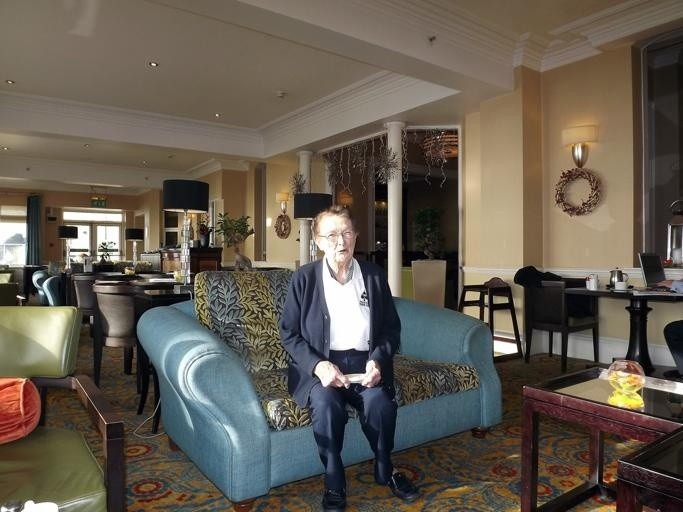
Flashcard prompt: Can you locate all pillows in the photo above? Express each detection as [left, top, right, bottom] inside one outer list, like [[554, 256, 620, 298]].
[[48, 259, 67, 276]]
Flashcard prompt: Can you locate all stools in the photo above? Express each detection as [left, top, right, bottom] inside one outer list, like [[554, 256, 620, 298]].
[[454, 283, 524, 364]]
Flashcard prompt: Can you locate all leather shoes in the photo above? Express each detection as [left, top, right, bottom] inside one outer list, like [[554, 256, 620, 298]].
[[322, 488, 347, 512], [388, 467, 421, 502]]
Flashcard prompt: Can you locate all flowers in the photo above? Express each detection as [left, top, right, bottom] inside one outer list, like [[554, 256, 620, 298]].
[[196, 210, 214, 235]]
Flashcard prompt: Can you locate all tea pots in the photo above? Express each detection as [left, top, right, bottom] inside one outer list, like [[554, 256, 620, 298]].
[[609, 267, 628, 287]]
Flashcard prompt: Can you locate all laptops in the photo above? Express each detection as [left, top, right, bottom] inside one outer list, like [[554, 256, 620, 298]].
[[637, 253, 670, 291]]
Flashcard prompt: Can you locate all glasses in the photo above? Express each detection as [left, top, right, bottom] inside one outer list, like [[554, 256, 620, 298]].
[[316, 230, 356, 239]]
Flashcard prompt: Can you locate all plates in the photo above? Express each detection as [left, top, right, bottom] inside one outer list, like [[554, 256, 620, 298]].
[[613, 290, 627, 293]]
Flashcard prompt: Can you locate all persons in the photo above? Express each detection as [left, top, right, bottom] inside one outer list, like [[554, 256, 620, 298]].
[[654, 280, 683, 381], [279, 204, 419, 511]]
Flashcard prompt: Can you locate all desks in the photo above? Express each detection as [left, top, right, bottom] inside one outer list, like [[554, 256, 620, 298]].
[[94, 277, 199, 309], [565, 284, 683, 374], [57, 271, 174, 306]]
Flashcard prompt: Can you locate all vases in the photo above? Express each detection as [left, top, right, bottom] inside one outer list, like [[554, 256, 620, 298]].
[[199, 229, 213, 246]]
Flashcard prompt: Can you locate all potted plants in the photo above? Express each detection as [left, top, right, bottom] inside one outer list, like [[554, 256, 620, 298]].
[[213, 212, 270, 271], [92, 240, 116, 266]]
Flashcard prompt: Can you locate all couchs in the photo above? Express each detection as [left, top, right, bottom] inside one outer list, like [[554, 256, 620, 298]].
[[135, 265, 504, 512]]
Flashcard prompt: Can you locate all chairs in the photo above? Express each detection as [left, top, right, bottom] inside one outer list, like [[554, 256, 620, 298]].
[[70, 275, 102, 334], [523, 280, 601, 369], [0, 305, 126, 512], [0, 269, 15, 283], [91, 264, 113, 272], [87, 279, 144, 389], [31, 269, 51, 306], [661, 318, 683, 376], [42, 275, 70, 307], [70, 263, 83, 274], [0, 283, 25, 306]]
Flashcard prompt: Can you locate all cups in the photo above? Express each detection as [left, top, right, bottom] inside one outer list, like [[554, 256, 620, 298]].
[[615, 282, 625, 290]]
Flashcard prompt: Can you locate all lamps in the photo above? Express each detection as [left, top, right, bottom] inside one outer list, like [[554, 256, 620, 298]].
[[162, 178, 209, 295], [294, 192, 336, 264], [57, 226, 78, 273], [125, 229, 144, 269], [558, 122, 598, 167], [277, 190, 290, 213]]
